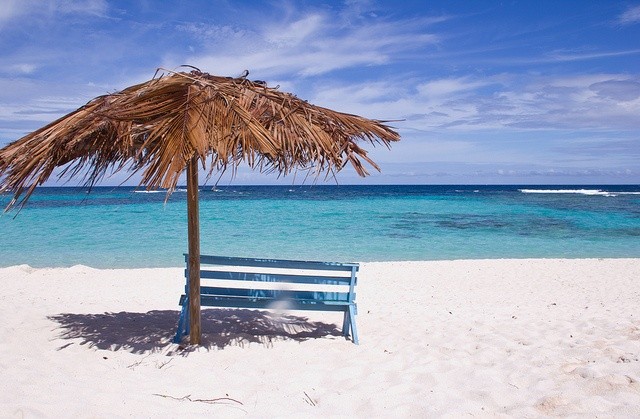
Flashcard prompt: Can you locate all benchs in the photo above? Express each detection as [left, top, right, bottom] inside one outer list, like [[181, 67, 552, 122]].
[[177, 253, 359, 345]]
